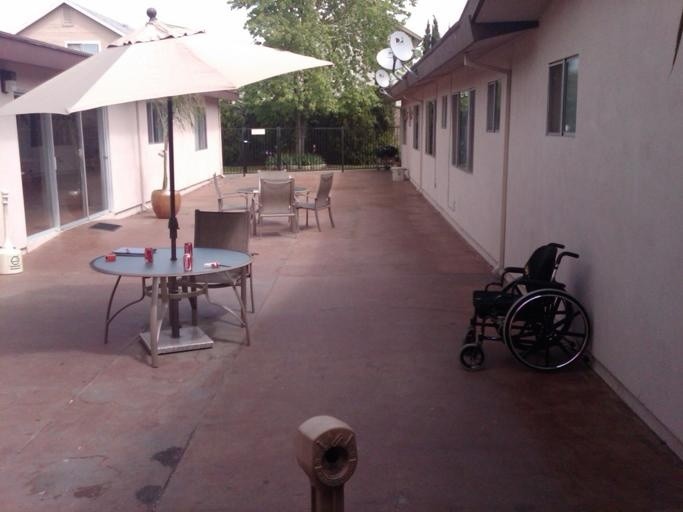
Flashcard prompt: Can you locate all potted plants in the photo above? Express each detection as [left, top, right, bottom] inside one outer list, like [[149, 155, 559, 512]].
[[149, 94, 205, 218]]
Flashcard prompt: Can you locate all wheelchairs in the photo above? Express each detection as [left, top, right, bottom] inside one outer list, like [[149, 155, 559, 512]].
[[456, 242, 592, 374]]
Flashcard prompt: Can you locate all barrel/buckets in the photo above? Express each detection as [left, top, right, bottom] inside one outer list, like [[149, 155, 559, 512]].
[[391, 167, 407, 181]]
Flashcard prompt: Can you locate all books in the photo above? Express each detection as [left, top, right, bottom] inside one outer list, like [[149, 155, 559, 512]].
[[111, 246, 155, 257]]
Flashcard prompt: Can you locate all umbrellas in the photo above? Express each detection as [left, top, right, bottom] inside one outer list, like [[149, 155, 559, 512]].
[[0, 6, 333, 339]]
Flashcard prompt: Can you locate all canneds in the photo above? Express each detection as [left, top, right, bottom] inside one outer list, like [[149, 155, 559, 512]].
[[144, 247, 153, 264], [184, 242, 192, 255], [183, 254, 192, 272]]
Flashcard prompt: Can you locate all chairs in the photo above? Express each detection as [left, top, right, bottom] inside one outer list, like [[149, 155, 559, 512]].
[[169, 209, 254, 346], [213, 168, 334, 235]]
